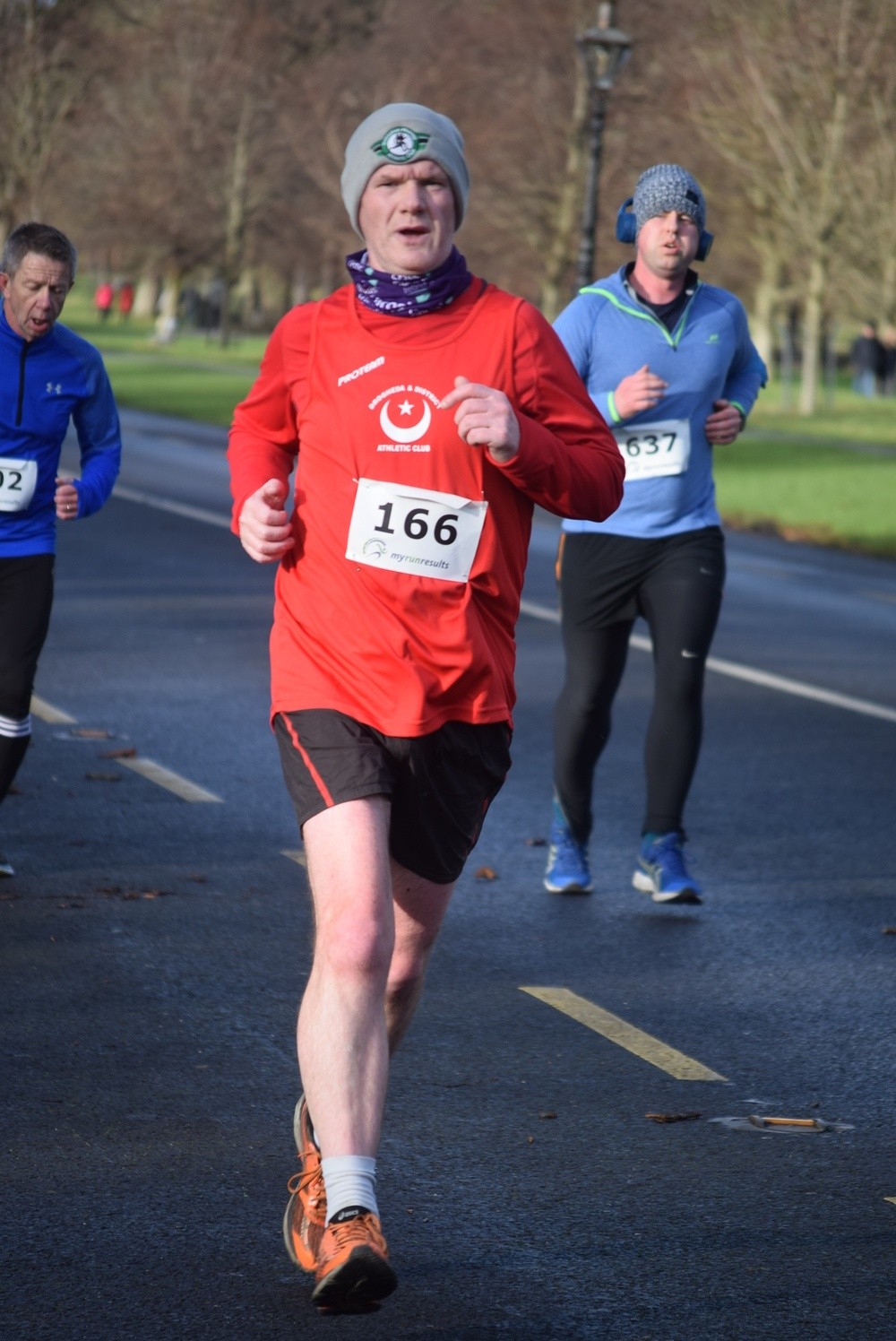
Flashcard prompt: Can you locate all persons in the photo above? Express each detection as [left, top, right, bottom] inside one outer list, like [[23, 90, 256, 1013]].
[[857, 319, 883, 397], [222, 104, 630, 1315], [119, 279, 134, 327], [94, 277, 114, 329], [542, 162, 771, 902], [0, 220, 126, 874]]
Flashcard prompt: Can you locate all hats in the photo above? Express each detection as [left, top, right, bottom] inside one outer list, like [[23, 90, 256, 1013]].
[[341, 103, 468, 238], [632, 164, 707, 237]]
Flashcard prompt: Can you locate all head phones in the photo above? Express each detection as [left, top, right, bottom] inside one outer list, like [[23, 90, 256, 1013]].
[[615, 198, 714, 261]]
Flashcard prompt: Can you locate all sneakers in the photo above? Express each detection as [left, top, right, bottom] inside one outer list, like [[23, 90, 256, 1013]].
[[311, 1213, 399, 1316], [283, 1092, 327, 1273], [543, 822, 594, 892], [631, 831, 703, 903]]
